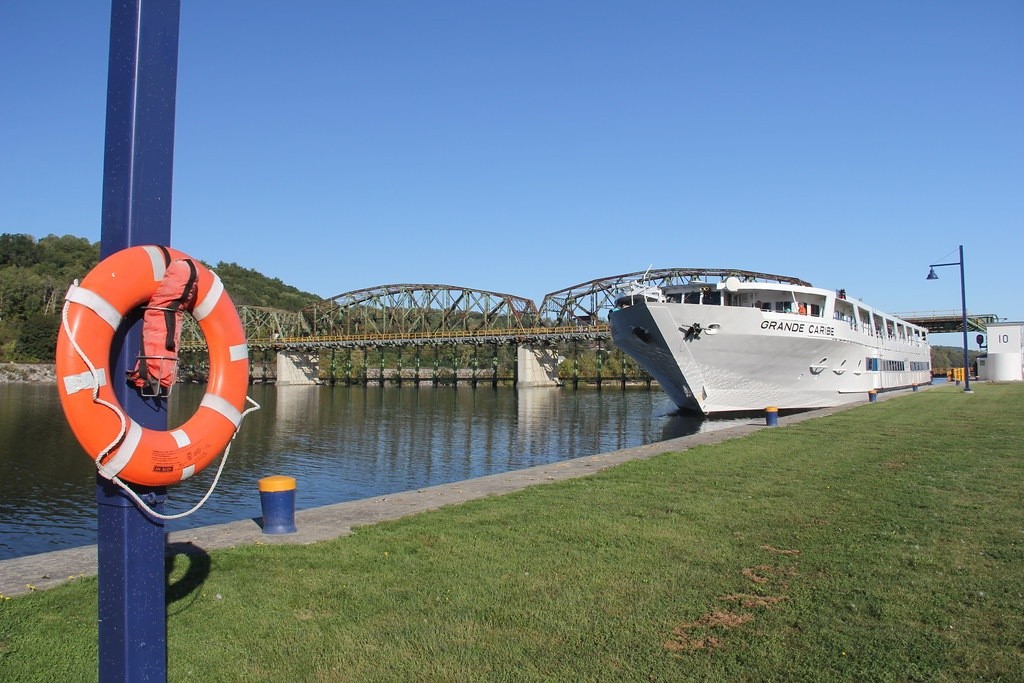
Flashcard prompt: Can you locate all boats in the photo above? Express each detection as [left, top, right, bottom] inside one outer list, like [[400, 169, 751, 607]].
[[608, 263, 933, 422]]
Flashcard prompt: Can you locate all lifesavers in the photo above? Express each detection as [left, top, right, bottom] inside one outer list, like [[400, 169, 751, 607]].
[[49, 243, 251, 490]]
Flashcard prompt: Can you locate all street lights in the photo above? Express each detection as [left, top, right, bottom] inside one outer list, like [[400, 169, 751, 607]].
[[926, 245, 974, 394]]
